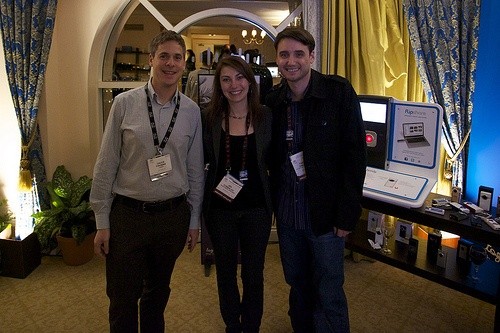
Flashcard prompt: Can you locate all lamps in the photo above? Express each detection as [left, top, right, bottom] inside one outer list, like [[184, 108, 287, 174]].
[[241, 29, 266, 45]]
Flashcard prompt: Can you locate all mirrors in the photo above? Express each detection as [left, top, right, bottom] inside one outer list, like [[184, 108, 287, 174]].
[[102, 0, 304, 130]]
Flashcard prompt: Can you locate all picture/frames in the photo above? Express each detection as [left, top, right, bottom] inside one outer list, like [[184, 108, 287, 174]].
[[197, 73, 263, 111]]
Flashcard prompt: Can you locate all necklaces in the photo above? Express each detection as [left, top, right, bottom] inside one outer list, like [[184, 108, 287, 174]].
[[230, 114, 247, 119]]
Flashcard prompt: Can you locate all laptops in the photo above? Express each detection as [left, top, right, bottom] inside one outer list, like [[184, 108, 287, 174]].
[[402, 123, 430, 148]]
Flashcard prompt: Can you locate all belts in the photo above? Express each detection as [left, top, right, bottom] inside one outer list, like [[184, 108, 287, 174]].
[[114, 194, 186, 214]]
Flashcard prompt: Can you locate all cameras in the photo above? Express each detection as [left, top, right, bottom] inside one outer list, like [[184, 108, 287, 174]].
[[436, 249, 448, 266], [375, 227, 385, 245], [371, 217, 378, 228], [400, 225, 406, 238], [459, 245, 467, 260], [408, 238, 418, 262], [425, 187, 500, 231]]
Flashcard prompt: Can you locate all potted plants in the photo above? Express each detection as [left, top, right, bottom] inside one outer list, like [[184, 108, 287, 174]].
[[29, 165, 96, 266]]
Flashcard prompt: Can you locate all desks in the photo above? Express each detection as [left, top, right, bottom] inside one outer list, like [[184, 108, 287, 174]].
[[344, 192, 500, 333]]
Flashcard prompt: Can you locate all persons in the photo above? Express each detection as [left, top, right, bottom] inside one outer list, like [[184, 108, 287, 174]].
[[269, 27, 367, 333], [185, 43, 262, 105], [89, 29, 203, 333], [202, 56, 280, 333]]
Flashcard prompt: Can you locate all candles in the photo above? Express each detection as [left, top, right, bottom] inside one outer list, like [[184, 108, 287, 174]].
[[238, 48, 242, 57], [215, 52, 218, 63], [207, 47, 211, 65], [246, 53, 249, 63]]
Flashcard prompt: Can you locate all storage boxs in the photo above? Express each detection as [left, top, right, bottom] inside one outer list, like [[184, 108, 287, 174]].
[[395, 219, 414, 244], [367, 210, 385, 233]]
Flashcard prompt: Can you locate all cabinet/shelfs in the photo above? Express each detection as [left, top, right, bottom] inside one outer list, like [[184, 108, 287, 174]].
[[114, 47, 151, 81]]
